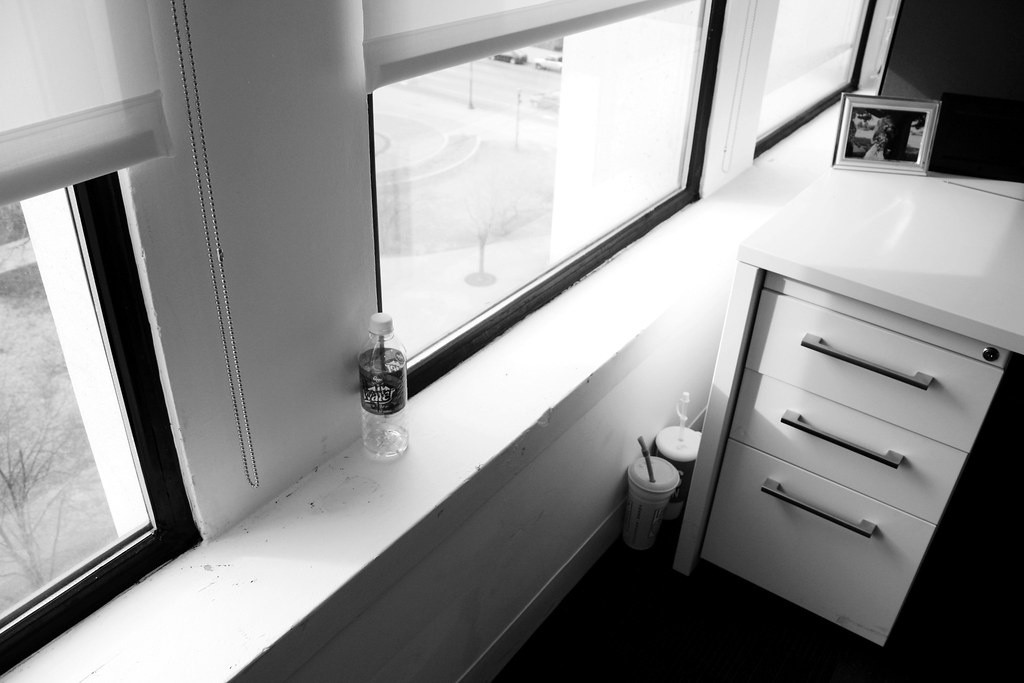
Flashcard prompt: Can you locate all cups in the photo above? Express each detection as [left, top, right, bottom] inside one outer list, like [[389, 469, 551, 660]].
[[624, 457, 680, 550], [656, 425, 701, 520]]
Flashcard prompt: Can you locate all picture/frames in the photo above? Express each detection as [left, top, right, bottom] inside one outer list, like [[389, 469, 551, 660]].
[[829, 92, 942, 178]]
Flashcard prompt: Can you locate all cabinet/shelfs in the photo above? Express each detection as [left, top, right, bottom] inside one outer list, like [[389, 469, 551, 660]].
[[669, 160, 1024, 647]]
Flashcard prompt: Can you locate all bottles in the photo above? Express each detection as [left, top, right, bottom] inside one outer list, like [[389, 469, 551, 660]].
[[359, 312, 407, 459]]
